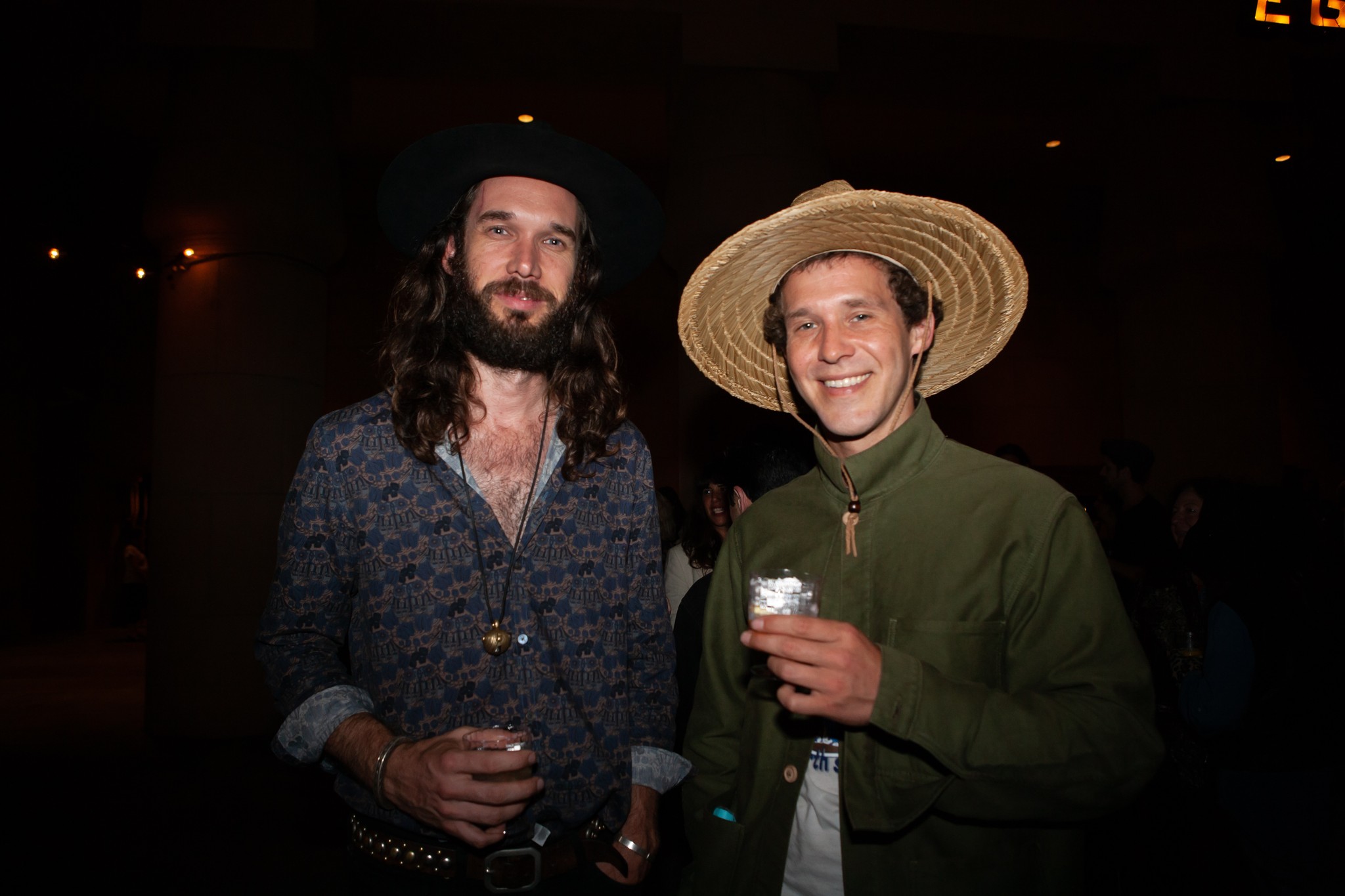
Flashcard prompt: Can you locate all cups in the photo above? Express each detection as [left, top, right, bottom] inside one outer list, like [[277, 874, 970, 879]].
[[461, 722, 535, 833], [749, 566, 822, 625]]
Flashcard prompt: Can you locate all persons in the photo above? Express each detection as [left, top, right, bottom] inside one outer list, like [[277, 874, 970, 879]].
[[641, 450, 811, 740], [254, 121, 694, 896], [119, 529, 149, 642], [993, 435, 1319, 850], [678, 178, 1165, 896]]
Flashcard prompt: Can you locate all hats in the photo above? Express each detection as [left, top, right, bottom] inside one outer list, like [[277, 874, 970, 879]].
[[677, 179, 1031, 414], [376, 121, 665, 298]]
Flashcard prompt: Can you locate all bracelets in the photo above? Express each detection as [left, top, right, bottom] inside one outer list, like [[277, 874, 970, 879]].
[[372, 735, 415, 810], [613, 833, 656, 864]]
